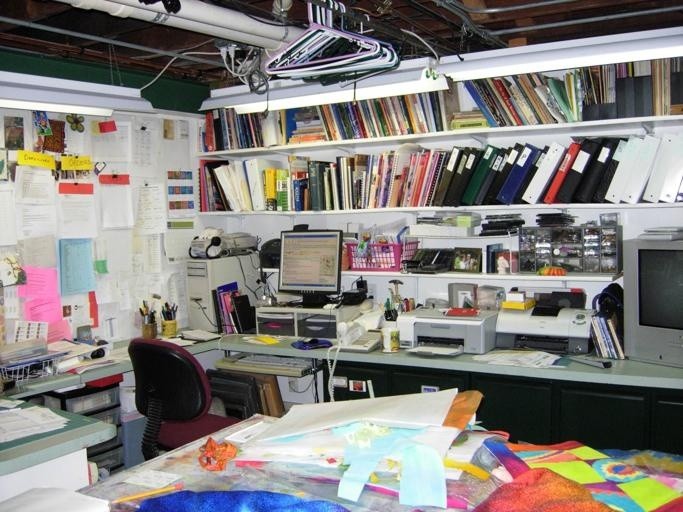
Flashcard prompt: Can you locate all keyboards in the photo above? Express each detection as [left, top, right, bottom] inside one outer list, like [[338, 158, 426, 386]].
[[237, 354, 315, 370]]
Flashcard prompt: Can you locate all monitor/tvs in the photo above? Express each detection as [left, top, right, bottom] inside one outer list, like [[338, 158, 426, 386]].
[[623, 239, 683, 368], [279, 229, 342, 307]]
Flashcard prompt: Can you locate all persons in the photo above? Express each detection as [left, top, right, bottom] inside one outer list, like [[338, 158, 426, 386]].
[[497, 255, 509, 274], [460, 254, 477, 270]]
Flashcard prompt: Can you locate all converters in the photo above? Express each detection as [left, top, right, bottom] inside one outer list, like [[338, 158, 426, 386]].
[[356, 280, 368, 292]]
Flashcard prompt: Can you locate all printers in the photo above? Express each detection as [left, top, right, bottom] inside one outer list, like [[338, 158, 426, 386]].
[[396, 308, 498, 356], [494, 291, 596, 355]]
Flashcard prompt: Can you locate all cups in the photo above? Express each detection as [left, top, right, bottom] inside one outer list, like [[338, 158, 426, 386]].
[[380, 320, 400, 353], [141, 324, 156, 340], [160, 320, 176, 339]]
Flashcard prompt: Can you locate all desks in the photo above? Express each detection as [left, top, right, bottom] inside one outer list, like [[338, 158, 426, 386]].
[[0, 328, 683, 502], [75, 413, 279, 512]]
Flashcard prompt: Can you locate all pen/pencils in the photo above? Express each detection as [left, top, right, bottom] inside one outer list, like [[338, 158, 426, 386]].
[[138, 298, 157, 324], [161, 301, 179, 321]]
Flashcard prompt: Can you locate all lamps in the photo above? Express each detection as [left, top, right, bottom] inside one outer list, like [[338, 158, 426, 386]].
[[0, 71, 159, 117]]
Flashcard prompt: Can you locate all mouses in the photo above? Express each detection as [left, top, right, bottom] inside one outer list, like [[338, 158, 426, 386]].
[[302, 337, 319, 344]]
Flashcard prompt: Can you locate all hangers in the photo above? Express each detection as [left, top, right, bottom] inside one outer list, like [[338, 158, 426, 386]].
[[267, 0, 399, 88]]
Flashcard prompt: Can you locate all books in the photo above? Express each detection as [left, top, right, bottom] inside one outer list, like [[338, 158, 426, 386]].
[[212, 283, 255, 334], [590, 312, 624, 361], [197, 55, 683, 153], [202, 145, 445, 210]]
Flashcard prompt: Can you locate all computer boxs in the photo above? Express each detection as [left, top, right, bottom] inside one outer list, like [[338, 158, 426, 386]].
[[186, 253, 262, 333]]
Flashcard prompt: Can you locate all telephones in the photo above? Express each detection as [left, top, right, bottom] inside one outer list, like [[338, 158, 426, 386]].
[[336, 324, 384, 352]]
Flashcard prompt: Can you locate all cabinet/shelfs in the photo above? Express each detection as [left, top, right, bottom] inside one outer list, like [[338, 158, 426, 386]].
[[197, 27, 683, 283]]
